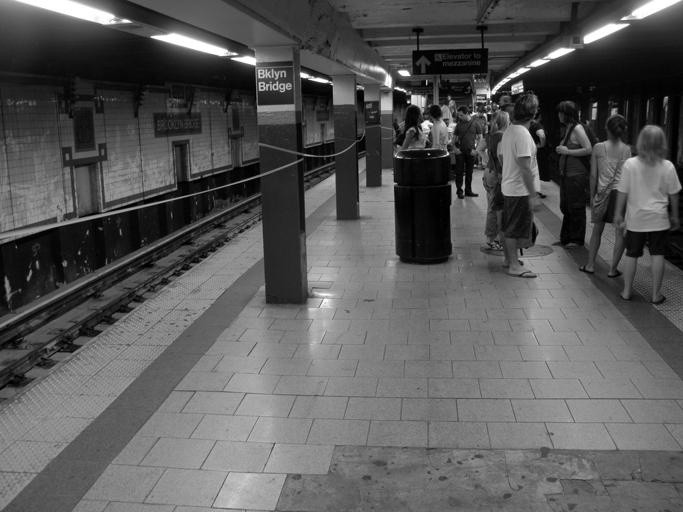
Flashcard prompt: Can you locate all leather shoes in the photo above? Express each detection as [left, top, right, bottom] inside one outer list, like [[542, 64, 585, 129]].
[[465, 192, 478, 197], [458, 193, 464, 199]]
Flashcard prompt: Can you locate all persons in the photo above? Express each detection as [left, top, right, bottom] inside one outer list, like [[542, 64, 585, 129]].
[[610, 124, 682, 304], [578, 114, 633, 278], [553, 100, 595, 251], [389, 91, 544, 280]]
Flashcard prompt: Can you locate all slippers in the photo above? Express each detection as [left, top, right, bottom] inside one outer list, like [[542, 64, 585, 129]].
[[502, 258, 523, 269], [620, 288, 632, 301], [579, 264, 595, 274], [507, 266, 537, 278], [608, 267, 621, 278], [651, 293, 666, 304]]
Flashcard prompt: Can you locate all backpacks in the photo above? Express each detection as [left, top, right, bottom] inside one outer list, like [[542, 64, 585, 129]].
[[566, 119, 601, 169]]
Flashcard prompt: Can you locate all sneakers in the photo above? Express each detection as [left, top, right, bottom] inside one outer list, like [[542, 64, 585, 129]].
[[484, 240, 504, 250]]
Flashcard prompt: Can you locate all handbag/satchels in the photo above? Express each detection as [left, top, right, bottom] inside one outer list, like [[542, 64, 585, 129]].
[[488, 184, 503, 211], [591, 192, 610, 221]]
